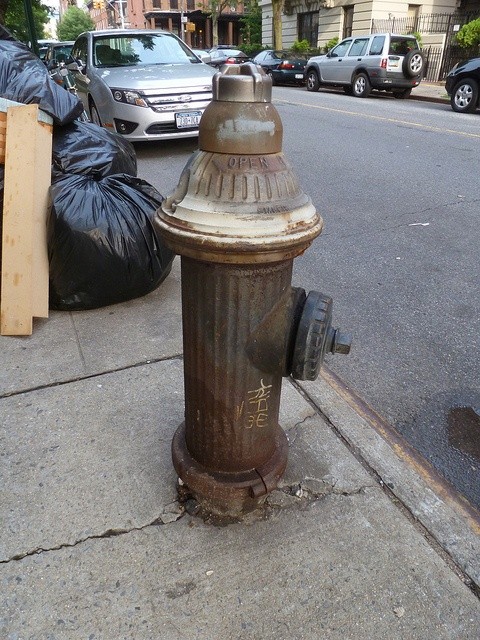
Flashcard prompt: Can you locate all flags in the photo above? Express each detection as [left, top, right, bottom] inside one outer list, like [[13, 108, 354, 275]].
[[92, 0, 106, 11]]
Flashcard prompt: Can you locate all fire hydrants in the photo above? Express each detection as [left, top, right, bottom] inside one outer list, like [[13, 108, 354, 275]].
[[152, 63, 354, 518]]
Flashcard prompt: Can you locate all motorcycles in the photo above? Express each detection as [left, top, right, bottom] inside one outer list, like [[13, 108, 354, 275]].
[[47, 53, 91, 124]]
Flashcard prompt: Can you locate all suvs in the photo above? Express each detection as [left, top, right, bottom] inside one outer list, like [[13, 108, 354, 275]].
[[302, 32, 426, 98]]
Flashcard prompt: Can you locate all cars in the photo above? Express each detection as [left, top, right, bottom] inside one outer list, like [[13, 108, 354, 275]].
[[245, 50, 309, 85], [445, 56, 480, 113], [61, 28, 220, 143], [44, 41, 81, 64], [203, 49, 251, 73]]
